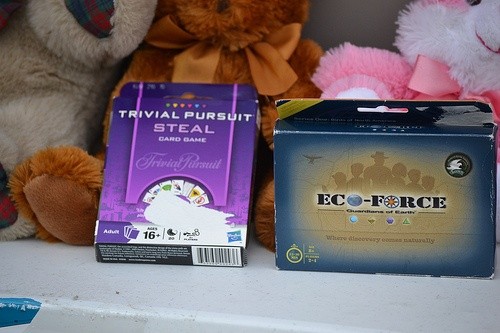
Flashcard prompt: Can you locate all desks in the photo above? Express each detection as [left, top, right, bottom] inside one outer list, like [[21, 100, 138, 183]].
[[0, 233, 500, 333]]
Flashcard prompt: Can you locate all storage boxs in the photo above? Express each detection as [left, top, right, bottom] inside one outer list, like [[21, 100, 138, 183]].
[[97, 82, 258, 268], [272, 97, 495, 278]]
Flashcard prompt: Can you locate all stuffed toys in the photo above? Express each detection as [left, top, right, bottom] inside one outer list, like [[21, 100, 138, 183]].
[[313, 0, 500, 244], [0, 0, 160, 242], [9, 0, 326, 251]]
